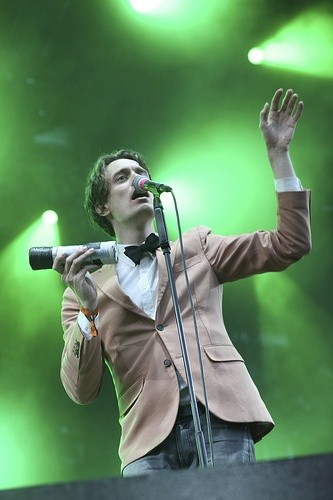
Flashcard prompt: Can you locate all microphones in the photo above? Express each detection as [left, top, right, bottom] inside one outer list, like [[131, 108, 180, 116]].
[[134, 176, 172, 194]]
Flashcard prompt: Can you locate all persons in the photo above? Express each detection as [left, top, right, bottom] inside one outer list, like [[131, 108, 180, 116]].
[[60, 88, 313, 476]]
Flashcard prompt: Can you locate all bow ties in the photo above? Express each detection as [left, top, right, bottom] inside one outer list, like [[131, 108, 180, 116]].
[[124, 233, 161, 264]]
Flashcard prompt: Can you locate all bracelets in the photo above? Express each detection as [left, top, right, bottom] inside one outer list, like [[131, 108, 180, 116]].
[[80, 307, 100, 338]]
